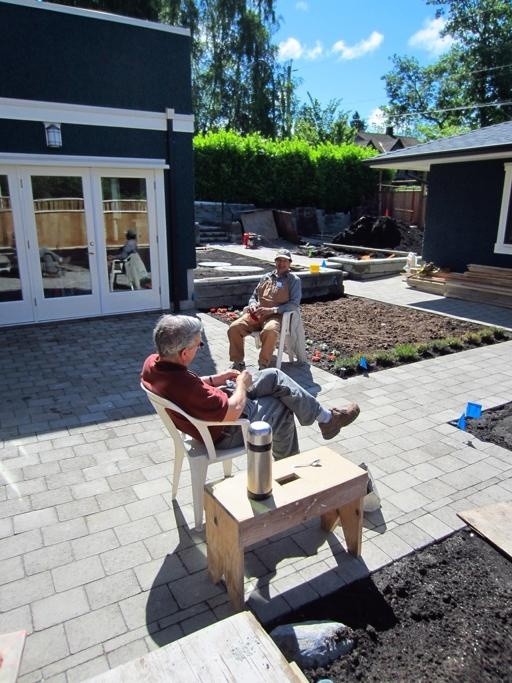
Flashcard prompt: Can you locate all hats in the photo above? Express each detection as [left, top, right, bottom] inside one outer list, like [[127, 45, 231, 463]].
[[274, 248, 293, 262]]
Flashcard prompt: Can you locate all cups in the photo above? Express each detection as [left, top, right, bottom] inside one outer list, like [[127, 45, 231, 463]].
[[309, 264, 319, 272]]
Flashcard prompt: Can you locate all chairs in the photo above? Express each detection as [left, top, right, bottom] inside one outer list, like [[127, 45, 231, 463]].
[[250, 307, 300, 369], [110, 254, 139, 291], [140, 381, 251, 532]]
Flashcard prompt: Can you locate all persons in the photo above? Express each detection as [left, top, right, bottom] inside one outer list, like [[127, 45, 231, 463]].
[[140, 313, 361, 463], [227, 248, 302, 371], [106, 229, 137, 273], [12, 232, 71, 277]]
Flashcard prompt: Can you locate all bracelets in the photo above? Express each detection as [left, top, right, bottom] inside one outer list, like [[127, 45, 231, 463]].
[[273, 307, 278, 314], [209, 375, 214, 387]]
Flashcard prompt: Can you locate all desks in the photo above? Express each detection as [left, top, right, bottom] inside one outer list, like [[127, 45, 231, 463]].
[[205, 446, 369, 610]]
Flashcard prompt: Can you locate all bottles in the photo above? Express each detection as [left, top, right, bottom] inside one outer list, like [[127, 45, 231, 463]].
[[322, 260, 327, 267], [246, 421, 273, 500]]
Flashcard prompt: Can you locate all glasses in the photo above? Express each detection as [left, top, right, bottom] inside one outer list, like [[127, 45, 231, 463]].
[[196, 340, 204, 349]]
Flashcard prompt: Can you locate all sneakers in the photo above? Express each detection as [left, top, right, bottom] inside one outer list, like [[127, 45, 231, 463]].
[[317, 402, 360, 440]]
